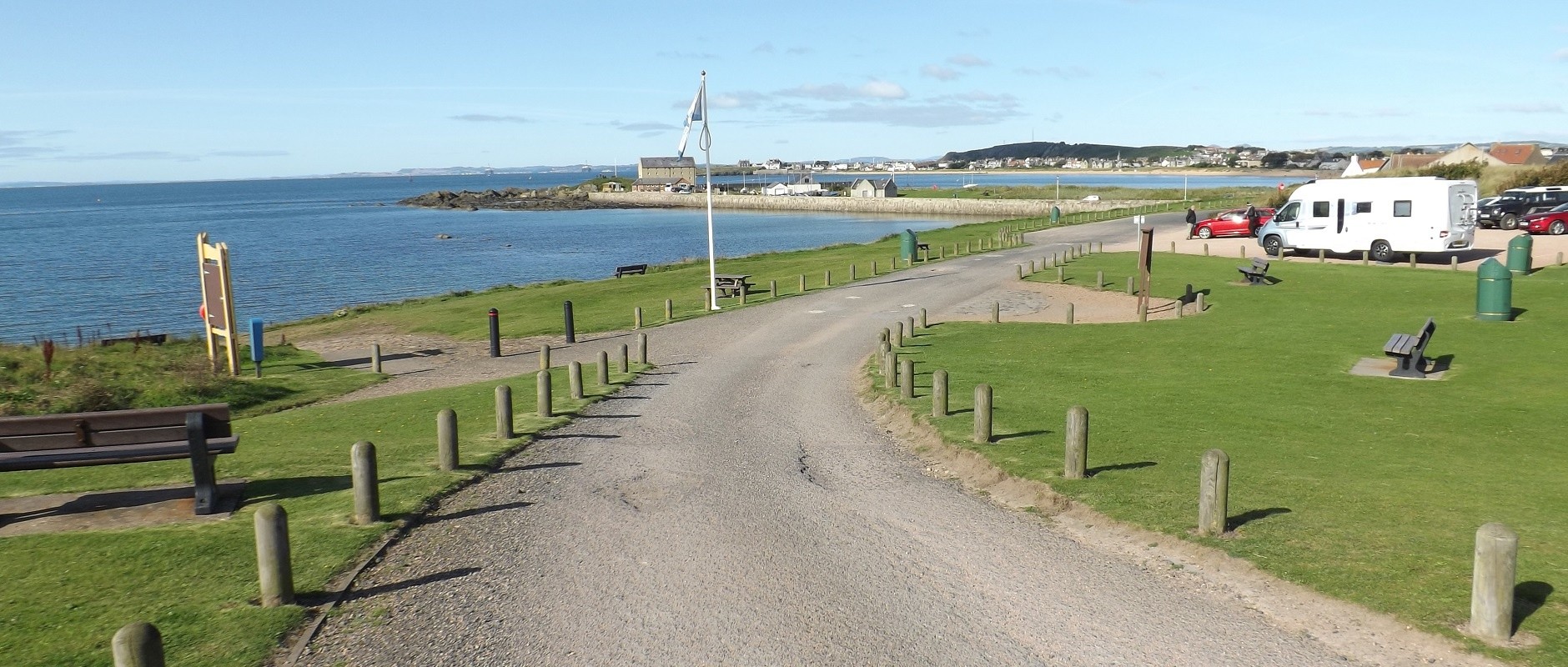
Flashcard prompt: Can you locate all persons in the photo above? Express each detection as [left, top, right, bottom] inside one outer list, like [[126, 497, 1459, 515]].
[[1185, 205, 1196, 240], [1243, 202, 1258, 238]]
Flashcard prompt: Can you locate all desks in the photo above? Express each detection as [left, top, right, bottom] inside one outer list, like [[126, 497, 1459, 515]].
[[714, 274, 751, 298]]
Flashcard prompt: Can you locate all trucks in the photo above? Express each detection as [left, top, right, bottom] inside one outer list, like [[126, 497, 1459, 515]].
[[1257, 175, 1479, 263]]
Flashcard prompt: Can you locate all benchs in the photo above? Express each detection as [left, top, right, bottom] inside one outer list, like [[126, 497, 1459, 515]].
[[0, 401, 238, 520], [614, 265, 647, 278], [101, 332, 165, 347], [1384, 318, 1435, 378], [918, 243, 930, 250], [1237, 257, 1270, 286], [701, 281, 755, 299]]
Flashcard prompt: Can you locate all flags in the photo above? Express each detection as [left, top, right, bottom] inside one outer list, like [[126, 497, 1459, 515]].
[[676, 80, 703, 160]]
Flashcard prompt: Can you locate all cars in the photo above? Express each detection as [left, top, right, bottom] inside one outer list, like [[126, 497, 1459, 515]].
[[1082, 195, 1101, 201], [1193, 207, 1278, 240], [1477, 195, 1524, 206], [1517, 202, 1568, 236]]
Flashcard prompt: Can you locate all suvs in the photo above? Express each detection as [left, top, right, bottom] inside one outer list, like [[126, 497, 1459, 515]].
[[1476, 185, 1568, 230]]
